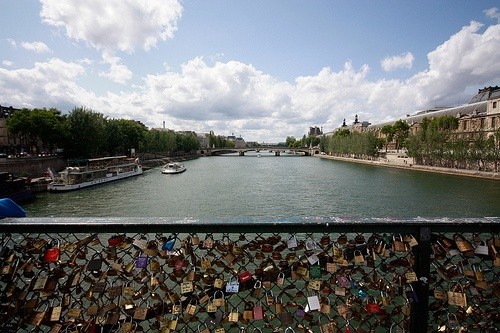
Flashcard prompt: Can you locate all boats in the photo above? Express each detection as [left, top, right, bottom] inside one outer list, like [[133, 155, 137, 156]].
[[161, 162, 187, 174], [0, 180, 39, 205], [46, 156, 143, 191]]
